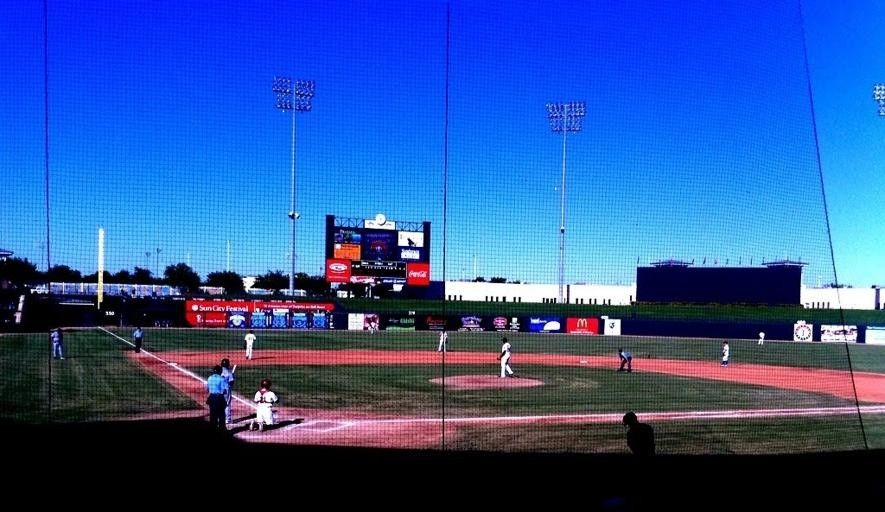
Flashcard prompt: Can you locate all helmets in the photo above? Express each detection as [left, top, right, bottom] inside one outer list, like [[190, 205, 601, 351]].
[[222, 358, 229, 367], [213, 365, 223, 374], [261, 380, 271, 387]]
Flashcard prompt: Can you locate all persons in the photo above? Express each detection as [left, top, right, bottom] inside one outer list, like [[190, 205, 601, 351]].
[[133, 324, 142, 351], [370, 320, 375, 333], [623, 411, 655, 456], [205, 357, 277, 443], [243, 330, 257, 363], [437, 326, 449, 352], [51, 326, 64, 359], [497, 336, 513, 379], [617, 347, 633, 371], [756, 330, 767, 346], [720, 340, 730, 366]]
